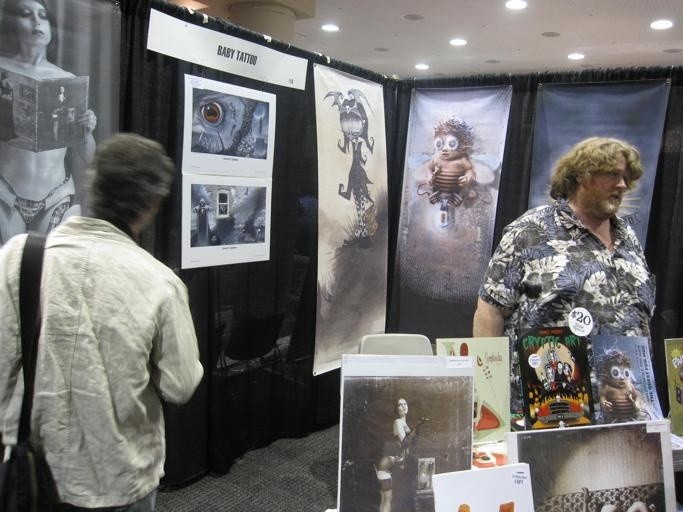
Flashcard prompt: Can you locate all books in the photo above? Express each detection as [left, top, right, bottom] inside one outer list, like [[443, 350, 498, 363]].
[[433, 333, 683, 448], [504, 418, 677, 512], [334, 352, 474, 512], [0, 68, 89, 154], [429, 462, 537, 512]]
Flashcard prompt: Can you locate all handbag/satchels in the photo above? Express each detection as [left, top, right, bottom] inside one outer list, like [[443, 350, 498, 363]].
[[1, 445, 60, 511]]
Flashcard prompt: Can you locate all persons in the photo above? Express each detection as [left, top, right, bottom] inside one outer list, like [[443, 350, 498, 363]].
[[422, 118, 476, 200], [470, 135, 659, 417], [49, 86, 68, 142], [375, 396, 428, 512], [0, 1, 96, 247], [191, 198, 214, 246], [2, 132, 209, 512]]
